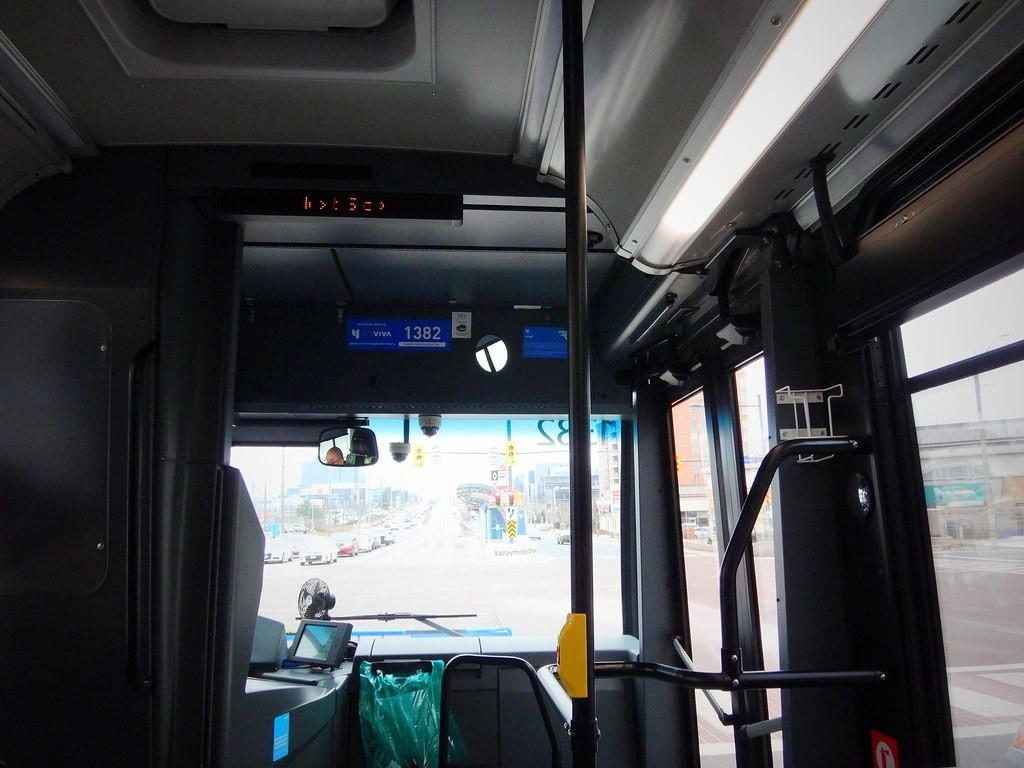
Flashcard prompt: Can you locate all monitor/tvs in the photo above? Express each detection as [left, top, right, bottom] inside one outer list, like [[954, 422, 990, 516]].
[[288, 620, 353, 670]]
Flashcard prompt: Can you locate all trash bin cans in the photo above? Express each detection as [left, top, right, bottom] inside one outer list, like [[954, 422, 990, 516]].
[[270, 523, 281, 538], [681, 524, 696, 540]]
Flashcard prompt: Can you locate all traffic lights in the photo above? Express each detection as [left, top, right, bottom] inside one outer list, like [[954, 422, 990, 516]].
[[505, 440, 518, 467], [413, 443, 425, 467]]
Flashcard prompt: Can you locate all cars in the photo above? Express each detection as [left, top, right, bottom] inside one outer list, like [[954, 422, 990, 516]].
[[557, 529, 569, 545], [693, 526, 710, 540], [934, 485, 978, 504], [263, 540, 293, 564], [527, 528, 541, 541], [298, 538, 338, 566], [284, 502, 433, 559]]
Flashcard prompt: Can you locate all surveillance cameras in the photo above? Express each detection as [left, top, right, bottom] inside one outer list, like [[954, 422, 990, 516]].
[[417, 415, 442, 437], [390, 443, 410, 463]]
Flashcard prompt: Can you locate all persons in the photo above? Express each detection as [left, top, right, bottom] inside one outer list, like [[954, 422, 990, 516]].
[[323, 447, 347, 464]]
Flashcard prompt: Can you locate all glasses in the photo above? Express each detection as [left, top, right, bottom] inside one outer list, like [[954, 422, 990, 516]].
[[324, 458, 342, 464]]
